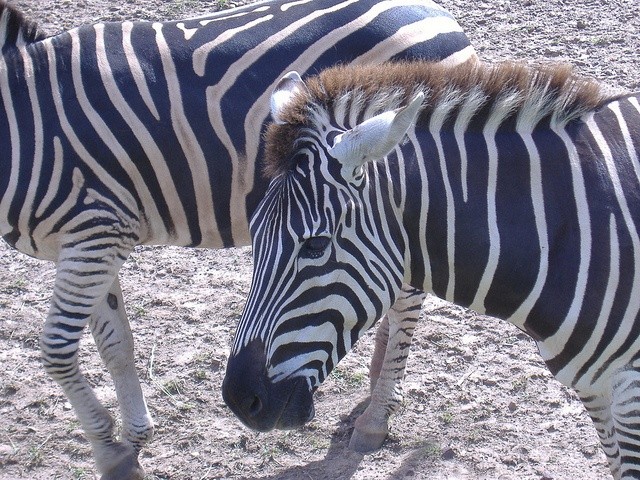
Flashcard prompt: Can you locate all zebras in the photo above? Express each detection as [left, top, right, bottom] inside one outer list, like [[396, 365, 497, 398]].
[[222, 57, 640, 480], [1, 1, 480, 479]]
